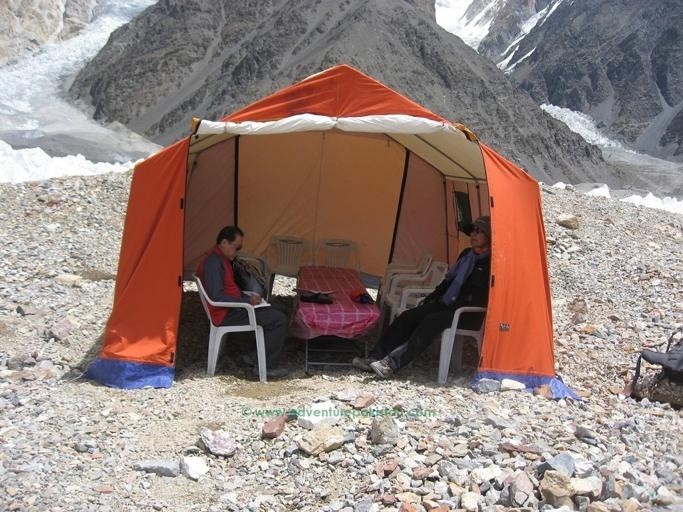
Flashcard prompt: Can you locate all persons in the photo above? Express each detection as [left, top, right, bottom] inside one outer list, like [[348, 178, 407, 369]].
[[352, 216, 490, 378], [196, 226, 289, 376]]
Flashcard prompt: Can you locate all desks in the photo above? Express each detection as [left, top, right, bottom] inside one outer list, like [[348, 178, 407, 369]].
[[292, 264, 382, 376]]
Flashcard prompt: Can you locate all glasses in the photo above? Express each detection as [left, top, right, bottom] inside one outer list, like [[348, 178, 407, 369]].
[[469, 227, 483, 233], [229, 242, 242, 251]]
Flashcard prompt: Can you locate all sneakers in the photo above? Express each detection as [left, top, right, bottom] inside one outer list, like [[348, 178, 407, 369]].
[[369, 356, 394, 378], [352, 354, 379, 373], [252, 365, 289, 378], [242, 354, 257, 367]]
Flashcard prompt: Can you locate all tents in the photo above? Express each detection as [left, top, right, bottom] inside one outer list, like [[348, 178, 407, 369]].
[[82, 64, 582, 400]]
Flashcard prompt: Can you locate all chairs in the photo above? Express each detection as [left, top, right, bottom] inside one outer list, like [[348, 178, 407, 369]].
[[193, 274, 268, 384], [259, 236, 306, 280], [379, 254, 487, 385], [314, 239, 362, 277]]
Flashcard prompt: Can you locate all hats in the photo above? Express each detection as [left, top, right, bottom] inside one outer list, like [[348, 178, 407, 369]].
[[462, 216, 490, 242]]
